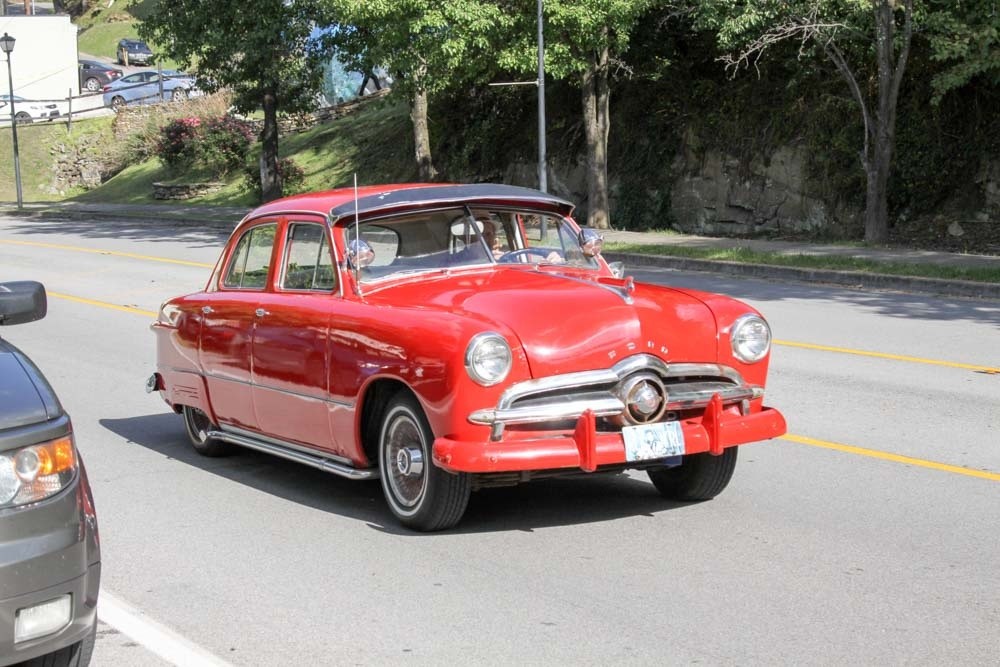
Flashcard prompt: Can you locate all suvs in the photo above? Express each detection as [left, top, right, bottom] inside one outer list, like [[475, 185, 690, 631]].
[[116, 39, 154, 67]]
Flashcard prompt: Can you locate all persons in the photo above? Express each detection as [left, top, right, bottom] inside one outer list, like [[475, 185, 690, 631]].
[[458, 210, 562, 264]]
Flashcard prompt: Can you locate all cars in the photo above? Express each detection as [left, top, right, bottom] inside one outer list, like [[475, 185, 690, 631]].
[[102, 69, 217, 114], [146, 182, 789, 534], [0, 280, 102, 667], [0, 94, 60, 125], [78, 59, 123, 92]]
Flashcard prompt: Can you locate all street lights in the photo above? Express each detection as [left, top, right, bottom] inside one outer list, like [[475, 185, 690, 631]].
[[0, 31, 23, 208]]
